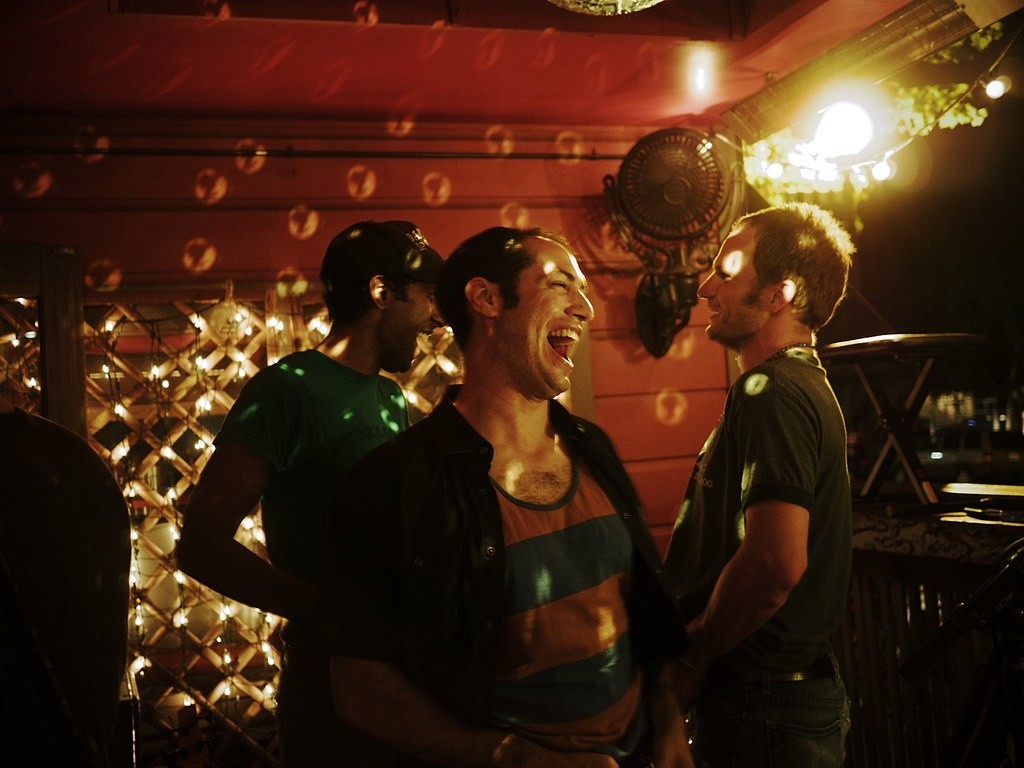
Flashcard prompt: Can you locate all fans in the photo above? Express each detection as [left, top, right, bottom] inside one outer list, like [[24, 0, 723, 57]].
[[600, 127, 738, 279]]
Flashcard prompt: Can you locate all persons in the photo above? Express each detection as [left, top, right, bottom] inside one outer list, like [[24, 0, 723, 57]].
[[174, 217, 447, 768], [319, 226, 693, 768], [0, 400, 133, 768], [662, 204, 852, 767]]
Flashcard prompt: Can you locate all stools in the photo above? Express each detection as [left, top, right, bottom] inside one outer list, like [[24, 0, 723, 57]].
[[812, 331, 979, 508]]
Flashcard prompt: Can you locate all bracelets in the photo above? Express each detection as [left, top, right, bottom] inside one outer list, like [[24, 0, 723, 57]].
[[489, 734, 519, 768]]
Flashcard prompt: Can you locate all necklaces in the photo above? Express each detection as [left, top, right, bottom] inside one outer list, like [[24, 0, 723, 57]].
[[765, 343, 810, 362]]
